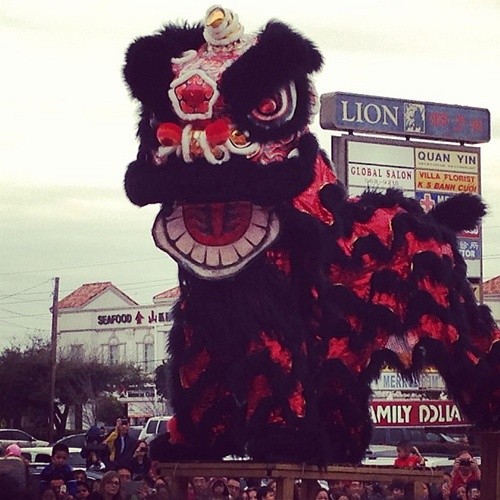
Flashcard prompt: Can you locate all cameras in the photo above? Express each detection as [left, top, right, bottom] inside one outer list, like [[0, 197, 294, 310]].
[[147, 489, 158, 496], [368, 482, 383, 495], [120, 419, 128, 424]]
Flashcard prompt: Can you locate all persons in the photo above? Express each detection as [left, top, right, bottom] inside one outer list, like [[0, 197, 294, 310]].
[[0, 415, 482, 500]]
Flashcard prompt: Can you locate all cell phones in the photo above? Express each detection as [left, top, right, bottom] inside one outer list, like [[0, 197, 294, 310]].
[[60, 484, 67, 495], [472, 488, 478, 499], [138, 447, 148, 452], [459, 458, 475, 466]]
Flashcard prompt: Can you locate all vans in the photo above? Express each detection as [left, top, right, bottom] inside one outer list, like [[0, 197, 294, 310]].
[[138, 415, 178, 444]]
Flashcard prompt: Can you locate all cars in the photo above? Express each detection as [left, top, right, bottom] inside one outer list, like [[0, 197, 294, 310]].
[[55, 433, 87, 448], [0, 428, 49, 450], [15, 446, 105, 474]]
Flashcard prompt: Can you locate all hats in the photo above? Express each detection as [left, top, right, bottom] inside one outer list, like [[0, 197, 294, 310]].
[[5, 444, 22, 456]]
